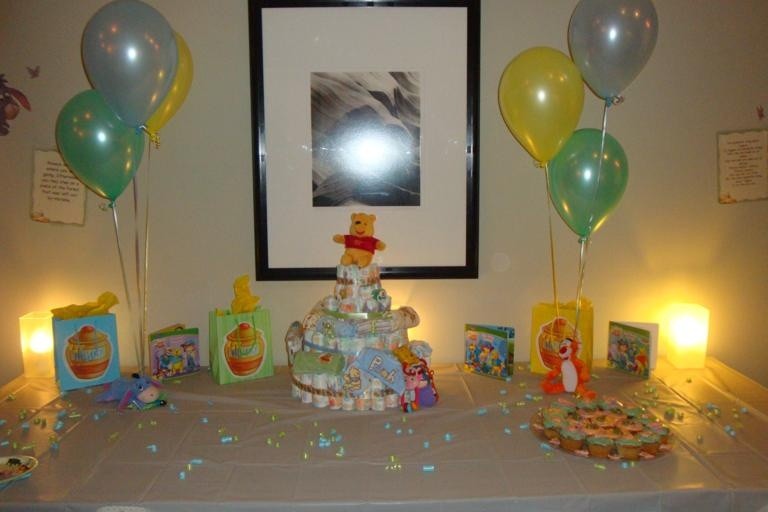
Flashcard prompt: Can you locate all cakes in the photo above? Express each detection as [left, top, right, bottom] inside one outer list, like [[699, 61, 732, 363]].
[[541, 396, 669, 460], [277, 263, 433, 412]]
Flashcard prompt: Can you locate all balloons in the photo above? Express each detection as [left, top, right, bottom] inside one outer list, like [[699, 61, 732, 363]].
[[54, 89, 145, 209], [80, 1, 180, 134], [144, 30, 194, 145], [547, 128, 629, 243], [567, 1, 660, 109], [498, 46, 586, 168]]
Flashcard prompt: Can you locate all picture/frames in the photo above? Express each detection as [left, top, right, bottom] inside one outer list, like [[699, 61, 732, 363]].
[[247, 0, 481, 283]]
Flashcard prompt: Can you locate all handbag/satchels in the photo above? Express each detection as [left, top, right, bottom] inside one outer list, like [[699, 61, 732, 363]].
[[53, 314, 121, 391], [530, 302, 594, 377], [209, 305, 274, 385]]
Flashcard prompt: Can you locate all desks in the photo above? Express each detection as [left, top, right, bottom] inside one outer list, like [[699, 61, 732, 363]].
[[0, 362, 768, 512]]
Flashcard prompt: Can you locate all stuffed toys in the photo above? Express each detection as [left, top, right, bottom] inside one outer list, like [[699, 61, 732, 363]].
[[540, 338, 597, 400], [332, 212, 385, 268], [94, 375, 166, 411], [393, 344, 439, 412]]
